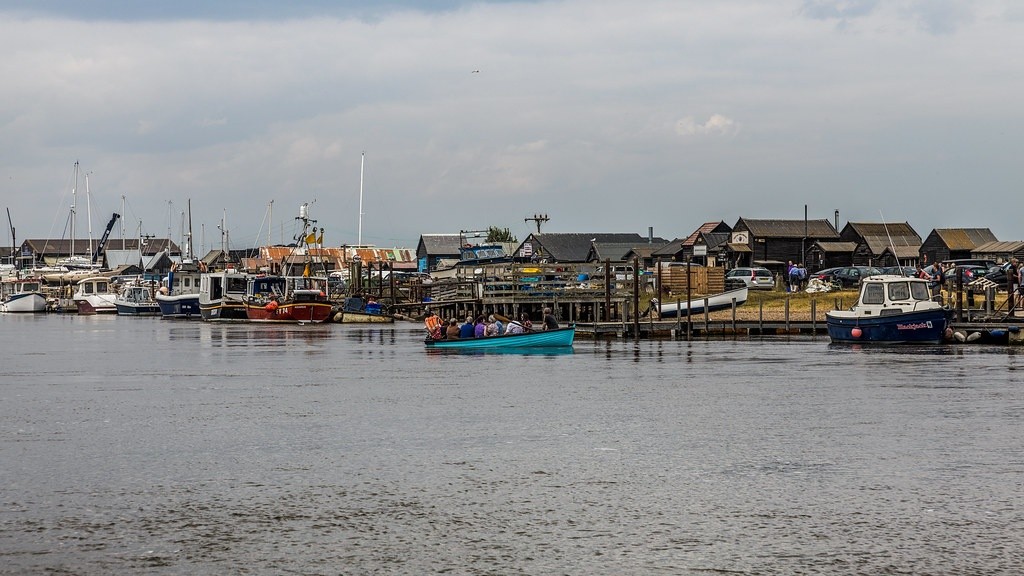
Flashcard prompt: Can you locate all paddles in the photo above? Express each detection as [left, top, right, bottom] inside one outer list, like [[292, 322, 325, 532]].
[[493, 313, 536, 331]]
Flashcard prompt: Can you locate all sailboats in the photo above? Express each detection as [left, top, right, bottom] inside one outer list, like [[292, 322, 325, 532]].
[[0, 161, 121, 316], [115, 194, 335, 323]]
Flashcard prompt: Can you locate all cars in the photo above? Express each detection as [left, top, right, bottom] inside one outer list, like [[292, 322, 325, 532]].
[[809, 259, 1016, 287]]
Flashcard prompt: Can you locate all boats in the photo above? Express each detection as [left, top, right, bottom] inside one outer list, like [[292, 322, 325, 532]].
[[333, 295, 396, 324], [826, 277, 954, 345], [424, 323, 576, 348], [654, 287, 748, 318]]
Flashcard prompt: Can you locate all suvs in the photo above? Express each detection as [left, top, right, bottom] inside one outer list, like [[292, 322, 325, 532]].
[[594, 266, 634, 281], [726, 266, 775, 290]]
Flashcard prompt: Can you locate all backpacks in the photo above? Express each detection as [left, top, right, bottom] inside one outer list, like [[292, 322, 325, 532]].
[[935, 271, 945, 284]]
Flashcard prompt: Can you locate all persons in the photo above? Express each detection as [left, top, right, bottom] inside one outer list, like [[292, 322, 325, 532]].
[[542, 308, 559, 330], [788, 260, 807, 292], [1004, 259, 1024, 307], [441, 313, 532, 339], [914, 262, 955, 302]]
